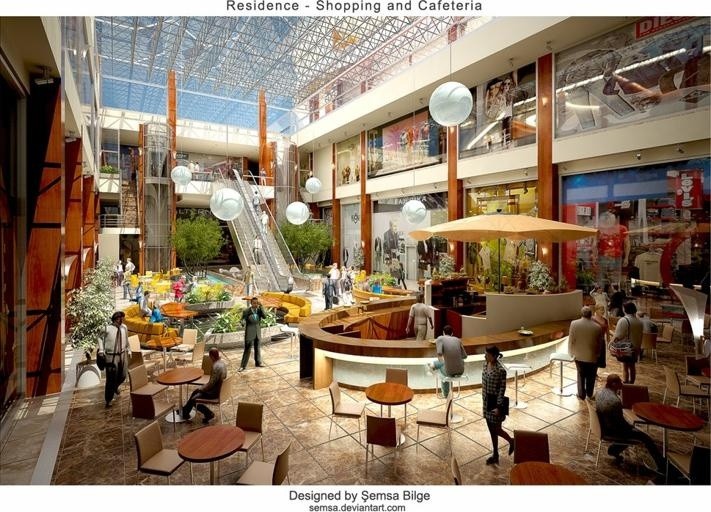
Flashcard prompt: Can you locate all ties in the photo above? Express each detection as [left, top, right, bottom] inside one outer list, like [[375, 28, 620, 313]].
[[117, 326, 123, 354]]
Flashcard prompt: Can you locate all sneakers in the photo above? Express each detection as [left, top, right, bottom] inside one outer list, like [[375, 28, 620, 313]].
[[427, 362, 437, 374], [201, 412, 216, 424], [176, 409, 191, 419], [106, 388, 120, 408]]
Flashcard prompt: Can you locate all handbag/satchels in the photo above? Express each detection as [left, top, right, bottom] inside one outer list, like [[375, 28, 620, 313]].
[[607, 342, 637, 363], [332, 296, 339, 304], [97, 347, 106, 371], [486, 392, 510, 416]]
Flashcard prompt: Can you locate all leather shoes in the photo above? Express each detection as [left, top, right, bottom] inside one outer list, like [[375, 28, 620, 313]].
[[255, 362, 266, 368], [486, 454, 499, 464], [508, 439, 514, 456], [238, 367, 245, 372]]
